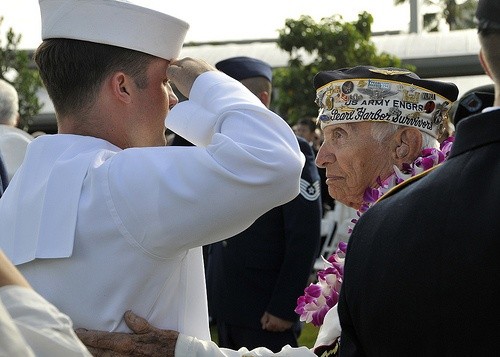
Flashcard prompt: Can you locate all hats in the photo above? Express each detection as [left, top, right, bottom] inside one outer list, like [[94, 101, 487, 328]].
[[476, 0, 500, 31], [449, 84, 495, 131], [38, 0, 190, 61], [215, 57, 273, 82], [312, 65, 458, 139]]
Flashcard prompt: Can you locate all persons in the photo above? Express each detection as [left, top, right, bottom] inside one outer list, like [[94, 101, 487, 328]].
[[0, 0, 500, 357], [0, 0, 306, 357]]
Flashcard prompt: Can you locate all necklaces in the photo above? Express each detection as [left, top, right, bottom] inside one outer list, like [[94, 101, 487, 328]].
[[295, 134, 454, 328]]
